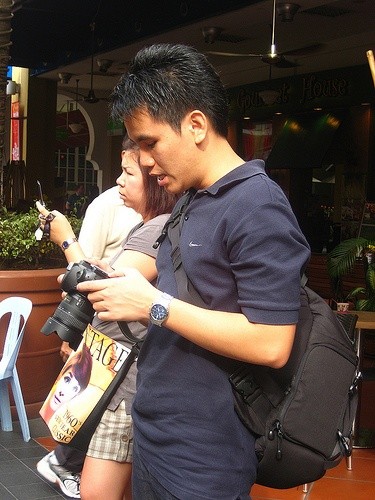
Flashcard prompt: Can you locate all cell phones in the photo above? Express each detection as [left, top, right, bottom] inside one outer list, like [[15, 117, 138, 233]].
[[37, 181, 45, 209]]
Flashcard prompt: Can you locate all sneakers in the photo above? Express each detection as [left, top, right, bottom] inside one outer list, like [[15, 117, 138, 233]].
[[36, 449, 81, 498]]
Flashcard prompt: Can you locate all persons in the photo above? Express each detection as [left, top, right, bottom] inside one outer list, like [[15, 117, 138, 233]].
[[40, 344, 92, 425], [58, 43, 311, 500], [66, 184, 84, 218], [81, 186, 99, 214], [34, 133, 186, 500], [36, 186, 143, 500]]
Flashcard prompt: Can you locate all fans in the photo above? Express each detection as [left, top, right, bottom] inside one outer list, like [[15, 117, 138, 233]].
[[58, 0, 356, 103]]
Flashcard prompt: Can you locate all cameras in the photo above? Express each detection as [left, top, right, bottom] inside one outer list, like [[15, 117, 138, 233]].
[[39, 261, 110, 353]]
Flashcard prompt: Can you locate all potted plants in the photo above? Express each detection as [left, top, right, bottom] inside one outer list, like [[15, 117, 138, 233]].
[[315, 234, 375, 429], [0, 200, 90, 421]]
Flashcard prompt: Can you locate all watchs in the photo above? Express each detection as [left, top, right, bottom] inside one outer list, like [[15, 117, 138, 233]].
[[62, 238, 77, 250], [149, 292, 174, 327]]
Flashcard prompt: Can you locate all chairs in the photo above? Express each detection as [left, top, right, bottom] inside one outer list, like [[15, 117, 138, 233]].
[[0, 297, 33, 443]]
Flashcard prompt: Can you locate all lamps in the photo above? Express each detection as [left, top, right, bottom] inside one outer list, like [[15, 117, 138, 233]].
[[4, 80, 18, 96], [68, 79, 84, 134]]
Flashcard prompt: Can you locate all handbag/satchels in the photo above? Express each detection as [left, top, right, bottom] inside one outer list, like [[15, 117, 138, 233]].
[[39, 323, 136, 448], [229, 271, 364, 489]]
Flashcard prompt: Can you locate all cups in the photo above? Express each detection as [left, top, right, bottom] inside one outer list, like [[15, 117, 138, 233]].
[[337, 303, 349, 312]]
